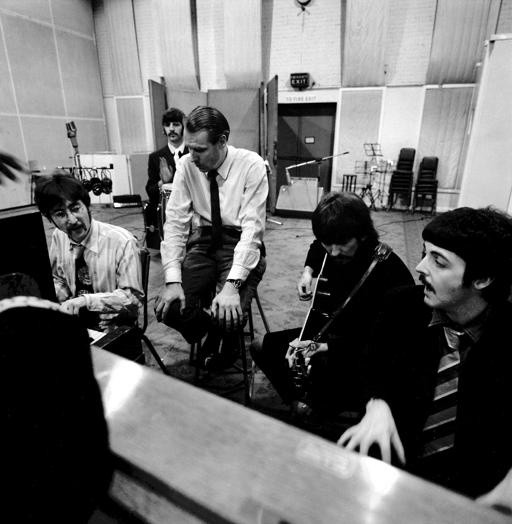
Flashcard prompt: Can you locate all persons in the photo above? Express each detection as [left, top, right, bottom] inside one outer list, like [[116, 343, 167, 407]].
[[150, 104, 269, 374], [261, 189, 422, 430], [141, 106, 190, 249], [31, 174, 148, 330], [337, 205, 512, 516]]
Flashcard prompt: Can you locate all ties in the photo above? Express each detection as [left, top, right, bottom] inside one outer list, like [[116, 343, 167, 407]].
[[174, 150, 180, 167], [420, 326, 463, 468], [74, 246, 98, 329], [207, 171, 222, 243]]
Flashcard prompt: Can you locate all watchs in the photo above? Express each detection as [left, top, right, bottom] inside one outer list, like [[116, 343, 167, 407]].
[[227, 278, 242, 288]]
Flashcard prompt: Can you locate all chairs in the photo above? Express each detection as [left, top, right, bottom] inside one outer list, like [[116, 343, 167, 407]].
[[179, 238, 271, 395], [410, 155, 439, 215], [98, 247, 167, 373], [386, 147, 415, 215]]
